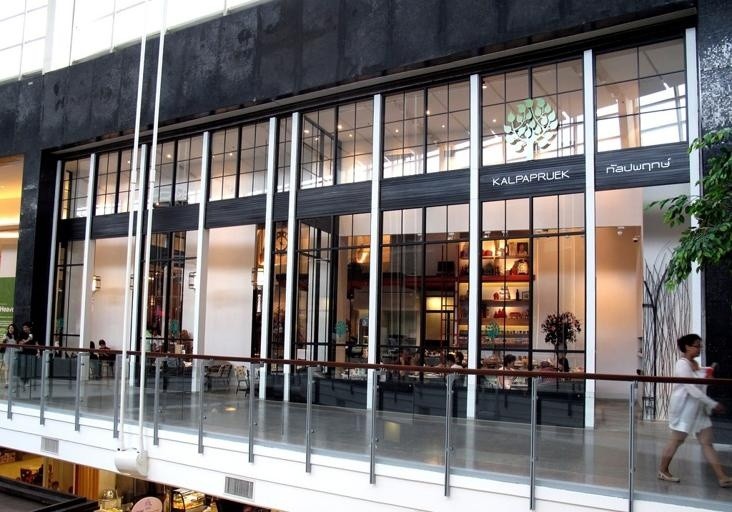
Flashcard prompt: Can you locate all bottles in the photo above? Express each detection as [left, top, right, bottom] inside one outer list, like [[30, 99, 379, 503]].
[[493, 308, 504, 318], [516, 289, 519, 299], [457, 329, 530, 346]]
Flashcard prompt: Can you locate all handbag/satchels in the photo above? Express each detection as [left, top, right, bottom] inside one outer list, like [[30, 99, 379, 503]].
[[0, 346, 6, 353]]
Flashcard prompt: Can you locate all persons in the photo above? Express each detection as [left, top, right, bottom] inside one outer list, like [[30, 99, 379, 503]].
[[68, 485, 73, 494], [55, 337, 59, 357], [557, 357, 574, 380], [19, 324, 41, 387], [98, 340, 111, 379], [146, 328, 192, 368], [519, 244, 527, 255], [49, 480, 60, 492], [22, 464, 43, 486], [540, 361, 557, 382], [3, 323, 23, 389], [395, 345, 466, 378], [90, 341, 97, 359], [657, 333, 732, 487], [498, 354, 516, 388]]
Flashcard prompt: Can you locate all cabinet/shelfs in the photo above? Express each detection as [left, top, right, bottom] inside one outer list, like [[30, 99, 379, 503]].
[[171, 488, 207, 511], [456, 241, 529, 347]]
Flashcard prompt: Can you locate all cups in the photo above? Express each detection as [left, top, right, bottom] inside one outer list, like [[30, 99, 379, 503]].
[[702, 365, 714, 380]]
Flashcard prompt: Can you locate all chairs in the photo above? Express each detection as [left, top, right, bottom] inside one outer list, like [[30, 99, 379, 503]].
[[209, 363, 250, 397]]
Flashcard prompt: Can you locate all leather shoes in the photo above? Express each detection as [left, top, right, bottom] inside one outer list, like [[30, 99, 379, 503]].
[[720, 477, 732, 487], [657, 470, 680, 483]]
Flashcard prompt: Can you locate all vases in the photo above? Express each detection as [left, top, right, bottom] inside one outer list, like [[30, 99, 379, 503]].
[[555, 343, 567, 359]]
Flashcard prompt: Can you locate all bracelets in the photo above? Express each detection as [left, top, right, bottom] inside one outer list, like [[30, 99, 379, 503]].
[[37, 351, 40, 353]]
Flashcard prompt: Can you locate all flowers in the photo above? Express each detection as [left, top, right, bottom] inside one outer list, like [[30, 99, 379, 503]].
[[541, 312, 581, 345]]
